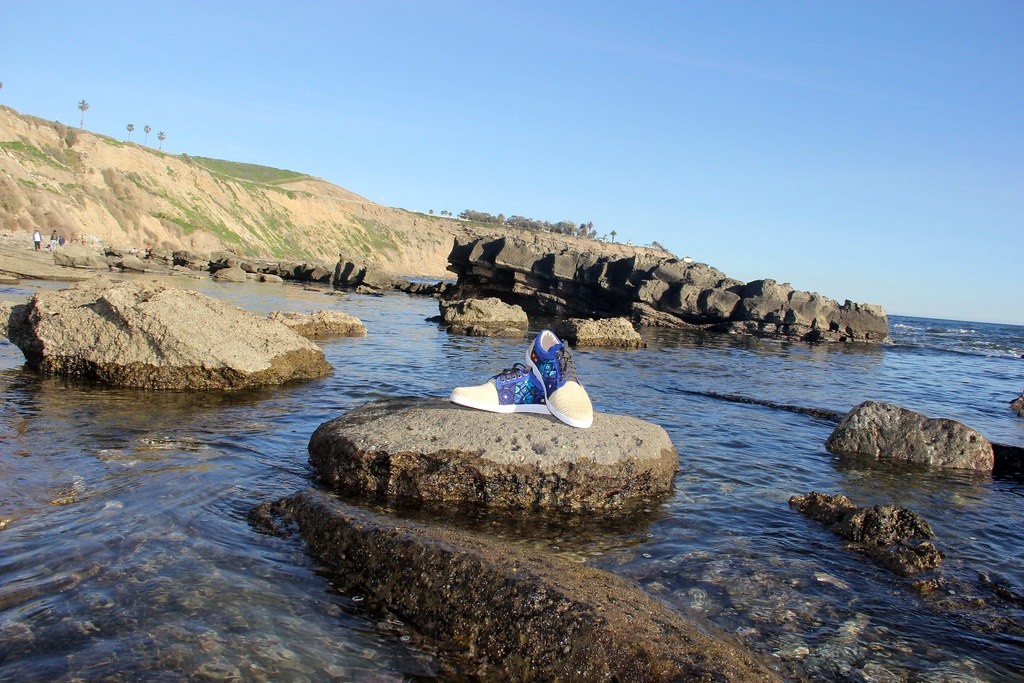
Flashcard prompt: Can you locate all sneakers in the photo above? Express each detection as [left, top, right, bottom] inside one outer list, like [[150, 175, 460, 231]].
[[450, 330, 593, 428]]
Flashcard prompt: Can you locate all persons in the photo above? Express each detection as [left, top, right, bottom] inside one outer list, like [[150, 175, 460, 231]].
[[146, 243, 153, 259], [59, 236, 65, 245], [33, 230, 42, 250], [49, 230, 58, 252]]
